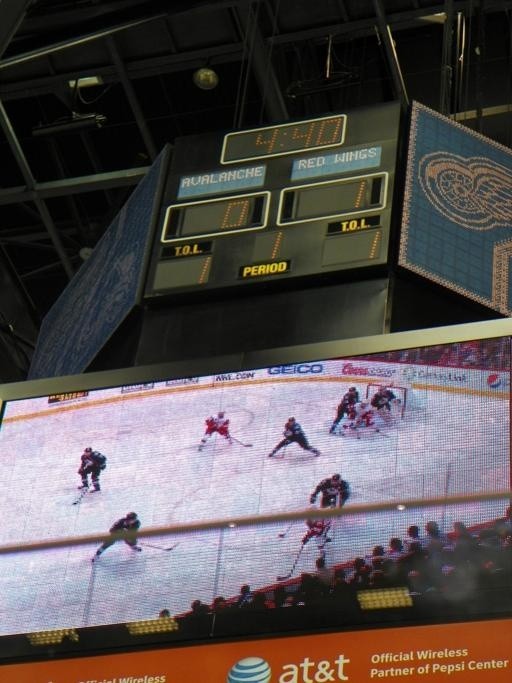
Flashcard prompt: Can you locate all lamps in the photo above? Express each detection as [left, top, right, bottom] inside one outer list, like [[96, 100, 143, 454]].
[[192, 55, 222, 92], [33, 76, 78, 116]]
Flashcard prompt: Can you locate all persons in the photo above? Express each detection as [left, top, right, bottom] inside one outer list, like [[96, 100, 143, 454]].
[[309, 473, 350, 509], [76, 445, 106, 492], [268, 419, 324, 458], [331, 384, 403, 432], [90, 510, 145, 558], [157, 507, 512, 619], [200, 409, 236, 452]]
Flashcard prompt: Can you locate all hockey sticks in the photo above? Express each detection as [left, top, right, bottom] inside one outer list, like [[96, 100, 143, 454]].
[[231, 437, 253, 447], [65, 477, 91, 507], [138, 543, 179, 551], [276, 542, 303, 581], [279, 520, 294, 537], [276, 441, 290, 458]]
